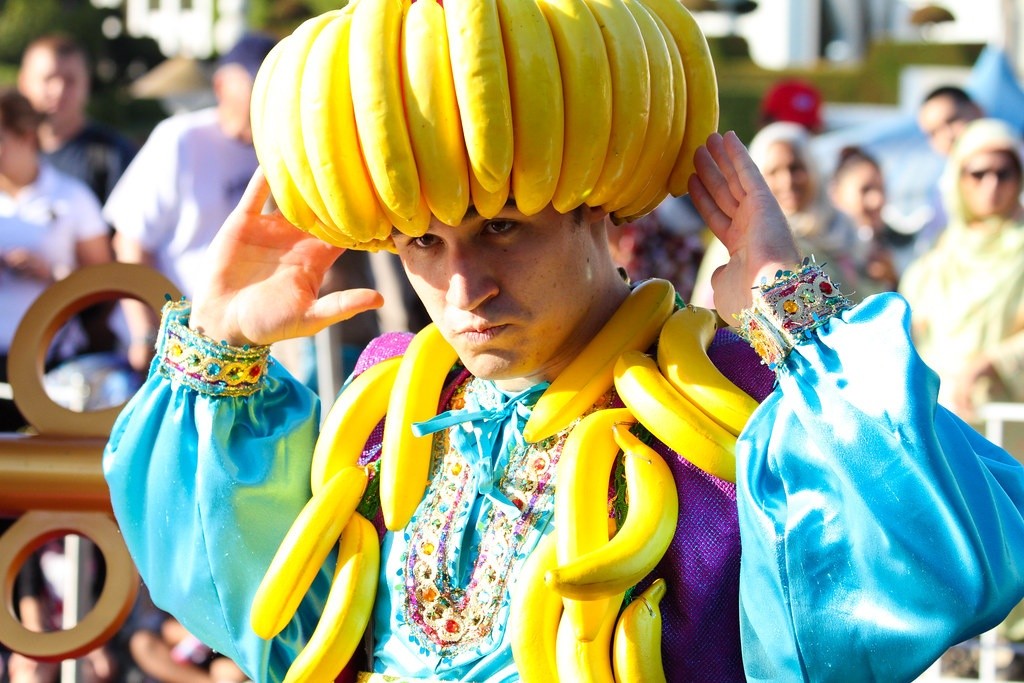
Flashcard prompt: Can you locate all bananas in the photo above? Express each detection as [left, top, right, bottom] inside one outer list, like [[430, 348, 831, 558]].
[[249, 277, 760, 683]]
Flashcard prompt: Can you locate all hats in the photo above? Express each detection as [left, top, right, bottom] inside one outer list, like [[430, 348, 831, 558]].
[[250, 0, 719, 255], [0, 90, 49, 136], [762, 79, 821, 130], [220, 34, 275, 76]]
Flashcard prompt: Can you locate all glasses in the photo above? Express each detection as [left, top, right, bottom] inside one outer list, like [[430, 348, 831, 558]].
[[968, 168, 1008, 183]]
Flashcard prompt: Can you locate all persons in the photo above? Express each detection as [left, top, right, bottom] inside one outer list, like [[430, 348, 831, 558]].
[[100, 0, 1024, 681], [101, 32, 317, 389], [897, 117, 1024, 681], [129, 586, 247, 682], [918, 87, 988, 161], [1, 516, 57, 682], [16, 30, 145, 356], [826, 144, 917, 291], [0, 89, 117, 431], [690, 122, 892, 309]]
